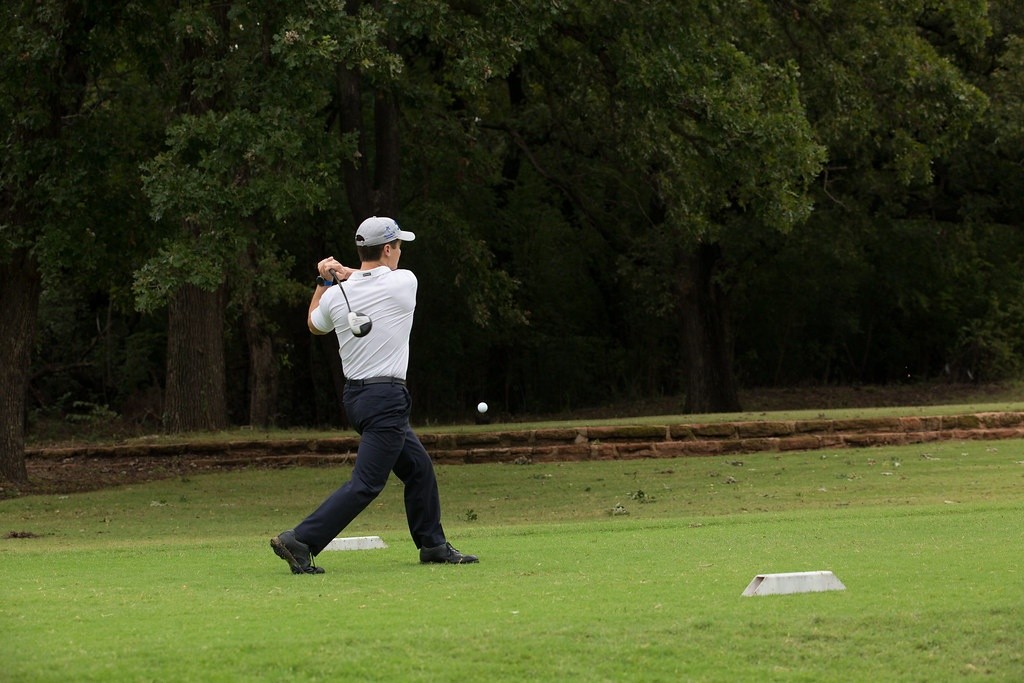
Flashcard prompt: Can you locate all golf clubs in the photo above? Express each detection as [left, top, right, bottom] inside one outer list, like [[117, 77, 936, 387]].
[[329, 268, 373, 337]]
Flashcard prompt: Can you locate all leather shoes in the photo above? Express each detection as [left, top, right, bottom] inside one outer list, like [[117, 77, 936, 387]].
[[420, 542, 479, 564], [270, 530, 325, 574]]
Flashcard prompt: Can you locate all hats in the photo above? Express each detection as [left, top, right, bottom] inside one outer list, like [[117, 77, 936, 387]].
[[355, 216, 416, 247]]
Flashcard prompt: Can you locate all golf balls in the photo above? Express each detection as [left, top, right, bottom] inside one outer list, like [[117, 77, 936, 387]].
[[478, 402, 488, 413]]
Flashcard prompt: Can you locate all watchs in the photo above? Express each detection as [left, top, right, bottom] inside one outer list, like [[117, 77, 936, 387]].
[[315, 275, 333, 287]]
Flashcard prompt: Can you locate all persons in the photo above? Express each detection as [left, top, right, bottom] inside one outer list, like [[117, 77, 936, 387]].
[[268, 216, 479, 575]]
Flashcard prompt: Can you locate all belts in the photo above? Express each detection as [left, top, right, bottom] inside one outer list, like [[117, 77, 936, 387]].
[[346, 377, 407, 386]]
